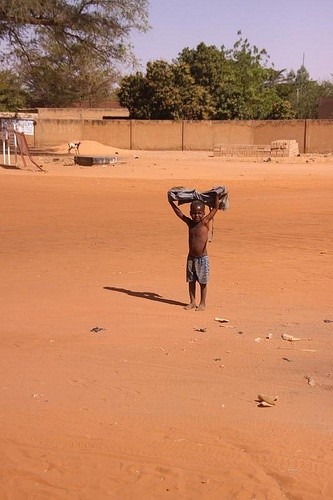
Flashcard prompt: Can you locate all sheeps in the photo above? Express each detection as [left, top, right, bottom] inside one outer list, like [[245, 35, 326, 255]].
[[68, 142, 81, 155]]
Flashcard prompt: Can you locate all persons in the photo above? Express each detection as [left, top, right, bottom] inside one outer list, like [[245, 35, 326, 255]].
[[167, 191, 219, 312]]
